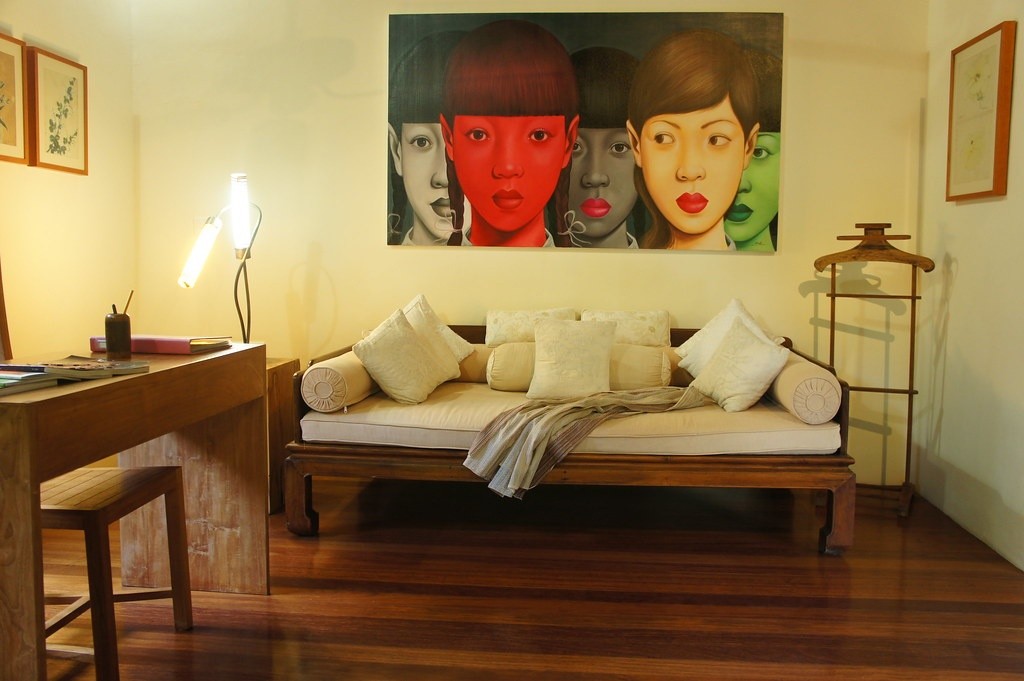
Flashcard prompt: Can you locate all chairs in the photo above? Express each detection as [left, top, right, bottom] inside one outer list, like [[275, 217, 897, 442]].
[[0, 266, 194, 681]]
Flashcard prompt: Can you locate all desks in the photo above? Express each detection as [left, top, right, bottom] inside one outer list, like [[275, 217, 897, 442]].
[[0, 342, 271, 681]]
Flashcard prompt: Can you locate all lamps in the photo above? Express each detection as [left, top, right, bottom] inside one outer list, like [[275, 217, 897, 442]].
[[180, 173, 263, 343]]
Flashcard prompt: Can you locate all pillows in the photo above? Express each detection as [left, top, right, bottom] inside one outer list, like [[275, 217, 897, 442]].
[[675, 297, 842, 424], [352, 294, 475, 404], [487, 341, 671, 390], [486, 309, 575, 345], [579, 306, 671, 348], [526, 320, 615, 398], [301, 350, 381, 413]]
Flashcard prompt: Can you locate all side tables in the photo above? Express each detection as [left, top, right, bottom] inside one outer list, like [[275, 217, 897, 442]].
[[266, 357, 301, 514]]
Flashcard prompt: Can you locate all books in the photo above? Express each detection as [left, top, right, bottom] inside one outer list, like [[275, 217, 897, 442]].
[[0, 354, 149, 395], [91, 334, 233, 355]]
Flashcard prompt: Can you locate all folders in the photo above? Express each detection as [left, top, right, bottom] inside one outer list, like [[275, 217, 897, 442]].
[[88, 334, 233, 355]]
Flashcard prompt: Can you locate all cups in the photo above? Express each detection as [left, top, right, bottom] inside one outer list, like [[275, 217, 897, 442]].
[[106, 313, 131, 360]]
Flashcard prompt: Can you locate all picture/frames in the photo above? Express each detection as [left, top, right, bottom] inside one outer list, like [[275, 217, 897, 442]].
[[0, 32, 30, 165], [26, 46, 89, 176], [946, 20, 1018, 200]]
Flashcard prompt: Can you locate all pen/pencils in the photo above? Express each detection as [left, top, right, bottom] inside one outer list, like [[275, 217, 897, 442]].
[[124, 290, 134, 314], [112, 304, 117, 314]]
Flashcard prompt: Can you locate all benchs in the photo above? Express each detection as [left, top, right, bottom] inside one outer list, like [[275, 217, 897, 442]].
[[284, 325, 857, 551]]
